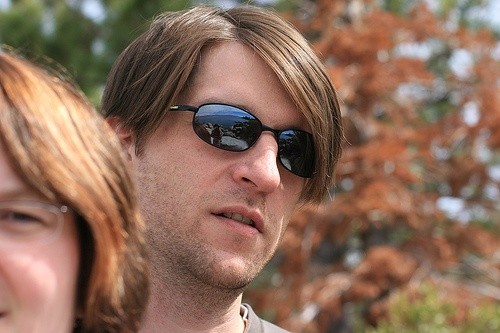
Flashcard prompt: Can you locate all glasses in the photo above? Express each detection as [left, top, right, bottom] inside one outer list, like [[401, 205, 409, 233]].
[[0, 199, 73, 250], [167, 102, 316, 178]]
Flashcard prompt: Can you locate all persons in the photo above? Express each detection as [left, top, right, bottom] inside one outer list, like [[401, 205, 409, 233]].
[[0, 45, 154, 333], [101, 5, 344, 333]]
